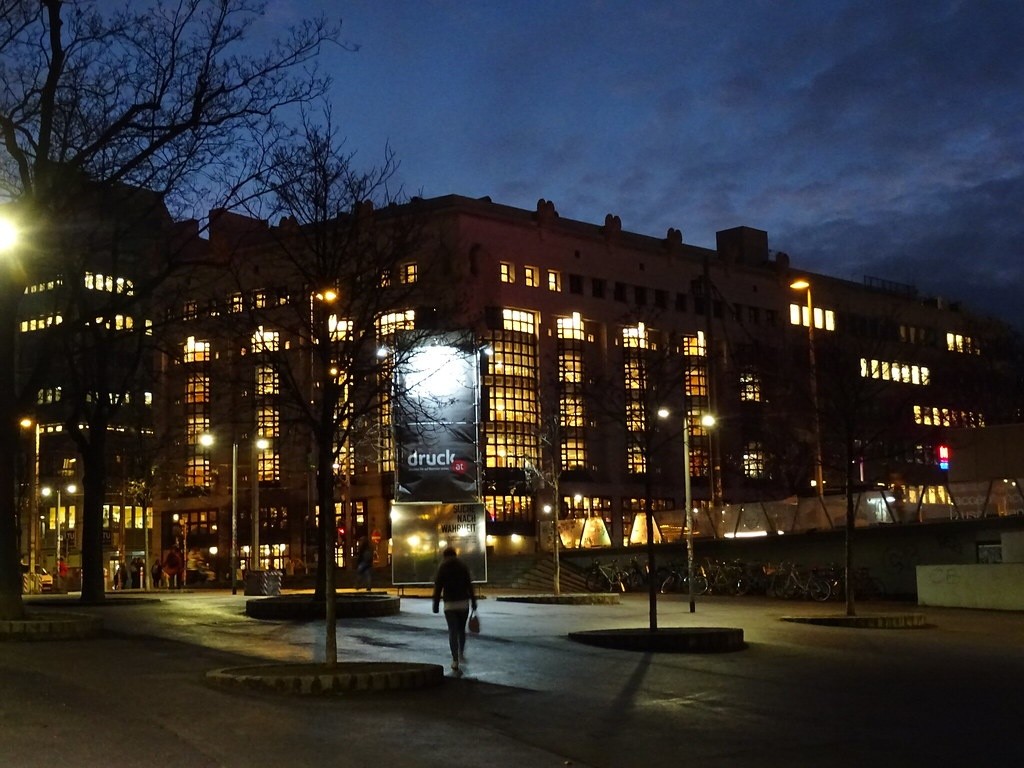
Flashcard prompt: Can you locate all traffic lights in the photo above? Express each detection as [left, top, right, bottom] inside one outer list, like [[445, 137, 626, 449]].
[[337, 528, 343, 547]]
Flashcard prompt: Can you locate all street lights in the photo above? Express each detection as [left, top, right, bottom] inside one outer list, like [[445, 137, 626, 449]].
[[309, 288, 335, 569], [658, 408, 715, 611], [201, 433, 271, 596], [41, 485, 78, 590], [21, 418, 38, 590], [791, 275, 822, 493], [173, 514, 187, 587]]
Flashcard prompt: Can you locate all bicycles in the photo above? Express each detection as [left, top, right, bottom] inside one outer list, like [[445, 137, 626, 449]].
[[585, 554, 843, 603]]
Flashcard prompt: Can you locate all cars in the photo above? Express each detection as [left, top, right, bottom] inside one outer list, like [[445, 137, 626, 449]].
[[22, 564, 52, 591]]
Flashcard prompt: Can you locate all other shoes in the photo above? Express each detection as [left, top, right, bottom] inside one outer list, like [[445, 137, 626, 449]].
[[451, 661, 459, 669], [459, 654, 464, 660]]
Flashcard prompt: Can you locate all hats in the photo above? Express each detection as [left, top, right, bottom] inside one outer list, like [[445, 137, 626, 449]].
[[443, 548, 456, 558]]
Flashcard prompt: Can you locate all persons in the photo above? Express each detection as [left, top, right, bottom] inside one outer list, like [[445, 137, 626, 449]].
[[433, 547, 476, 671], [130, 557, 146, 588], [352, 535, 372, 590], [151, 560, 162, 588]]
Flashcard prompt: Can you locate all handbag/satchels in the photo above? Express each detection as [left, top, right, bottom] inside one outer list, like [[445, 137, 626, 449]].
[[468, 610, 479, 633]]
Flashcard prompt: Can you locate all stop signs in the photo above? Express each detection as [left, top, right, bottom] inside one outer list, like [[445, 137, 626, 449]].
[[371, 530, 380, 543]]
